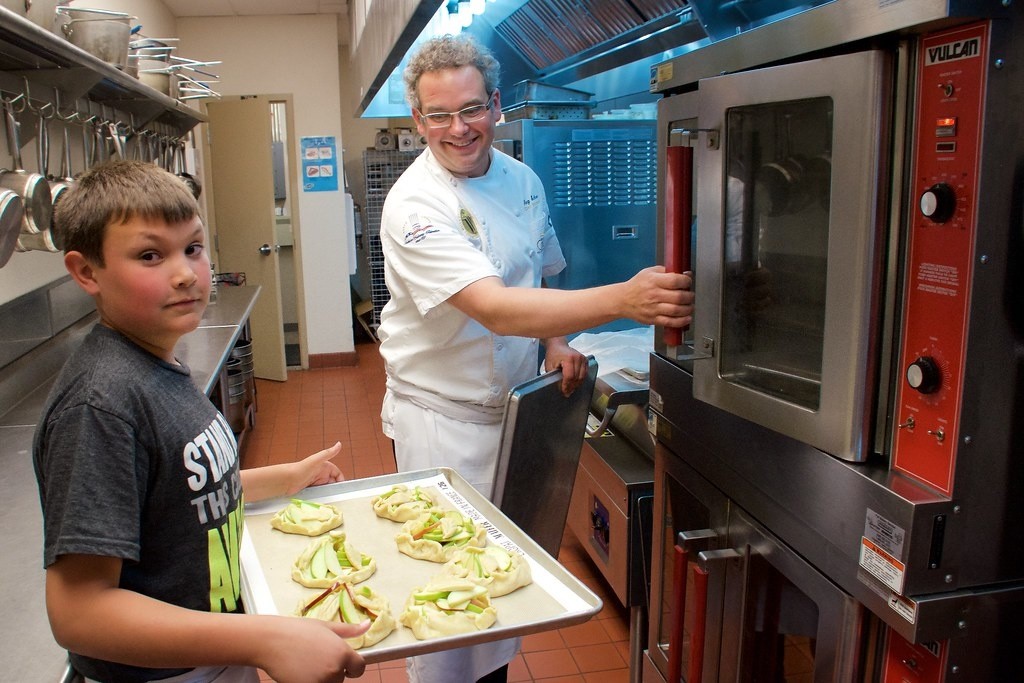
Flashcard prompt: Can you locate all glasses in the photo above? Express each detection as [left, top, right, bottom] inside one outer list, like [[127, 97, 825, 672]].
[[416, 93, 495, 129]]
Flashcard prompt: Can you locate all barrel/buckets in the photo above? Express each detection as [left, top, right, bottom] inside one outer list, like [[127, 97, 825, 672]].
[[226, 336, 255, 434]]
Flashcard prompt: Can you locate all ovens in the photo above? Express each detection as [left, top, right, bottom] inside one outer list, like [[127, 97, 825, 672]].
[[644, 2, 1024, 680]]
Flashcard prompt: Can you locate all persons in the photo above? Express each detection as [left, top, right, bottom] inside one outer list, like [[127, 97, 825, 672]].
[[28, 156, 370, 683], [376, 32, 700, 683]]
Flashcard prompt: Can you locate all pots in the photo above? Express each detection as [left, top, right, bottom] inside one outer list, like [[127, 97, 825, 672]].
[[1, 109, 79, 268], [0, 0, 224, 116]]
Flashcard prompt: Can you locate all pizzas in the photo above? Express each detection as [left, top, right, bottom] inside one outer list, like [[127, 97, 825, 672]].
[[271, 498, 397, 651], [370, 483, 534, 643]]
